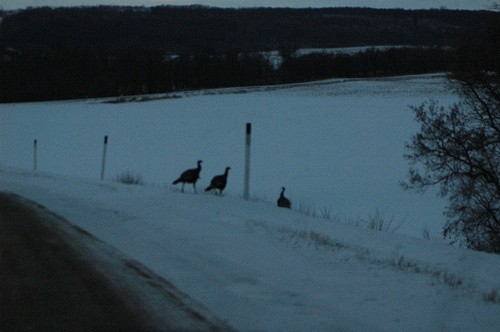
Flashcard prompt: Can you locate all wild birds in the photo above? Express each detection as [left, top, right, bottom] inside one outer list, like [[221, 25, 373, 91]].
[[276, 186, 291, 208], [173, 160, 202, 193], [205, 167, 231, 196]]
